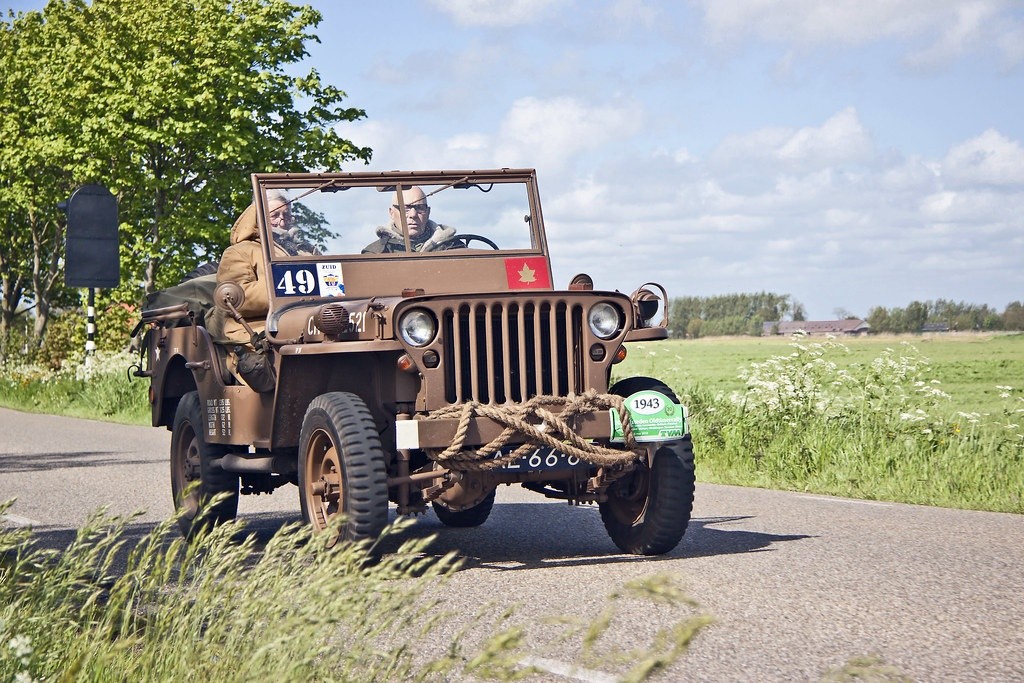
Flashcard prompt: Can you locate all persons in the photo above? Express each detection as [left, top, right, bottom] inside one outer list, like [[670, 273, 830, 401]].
[[203, 188, 323, 386], [360, 185, 467, 254]]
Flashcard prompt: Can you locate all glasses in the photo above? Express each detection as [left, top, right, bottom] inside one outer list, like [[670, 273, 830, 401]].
[[393, 204, 429, 213], [268, 214, 296, 225]]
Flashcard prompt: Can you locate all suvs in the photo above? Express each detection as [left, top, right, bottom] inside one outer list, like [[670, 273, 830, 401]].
[[125, 166, 698, 564]]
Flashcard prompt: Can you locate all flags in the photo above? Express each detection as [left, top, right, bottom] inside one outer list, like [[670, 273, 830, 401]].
[[503, 256, 549, 288]]
